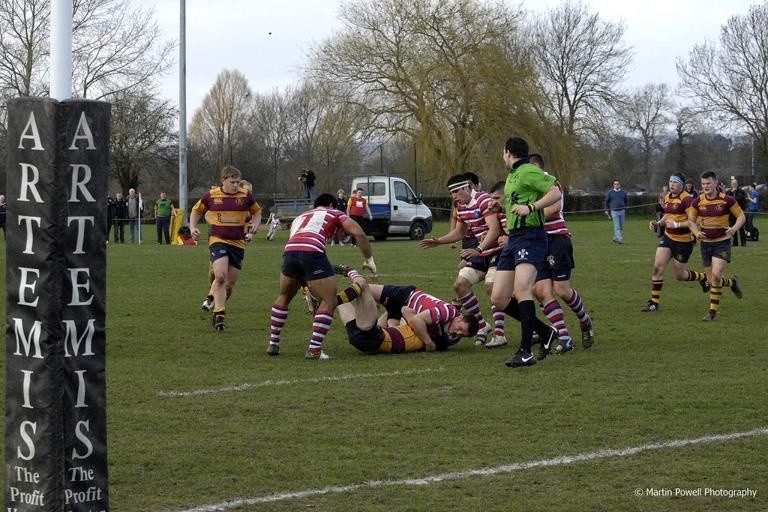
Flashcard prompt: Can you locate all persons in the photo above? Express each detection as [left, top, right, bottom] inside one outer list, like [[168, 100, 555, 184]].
[[203, 178, 254, 289], [636, 166, 763, 321], [255, 132, 595, 368], [604, 180, 627, 246], [188, 165, 263, 333], [153, 191, 179, 246], [0, 194, 8, 241], [110, 192, 127, 243], [123, 187, 146, 243], [104, 193, 115, 245]]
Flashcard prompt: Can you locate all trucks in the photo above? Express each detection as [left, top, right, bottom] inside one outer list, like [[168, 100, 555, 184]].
[[273, 172, 432, 241]]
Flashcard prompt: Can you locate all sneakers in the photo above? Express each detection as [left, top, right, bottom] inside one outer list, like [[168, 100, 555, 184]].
[[701, 313, 717, 321], [700, 279, 709, 293], [730, 274, 743, 299], [485, 334, 507, 347], [202, 298, 213, 311], [212, 314, 226, 333], [265, 343, 280, 356], [305, 349, 330, 360], [640, 301, 658, 312], [505, 348, 536, 366], [582, 315, 594, 348], [537, 325, 559, 360], [550, 338, 576, 354], [474, 322, 491, 347], [331, 263, 353, 277]]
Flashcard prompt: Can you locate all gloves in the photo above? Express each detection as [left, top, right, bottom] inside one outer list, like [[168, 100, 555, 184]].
[[363, 256, 377, 277]]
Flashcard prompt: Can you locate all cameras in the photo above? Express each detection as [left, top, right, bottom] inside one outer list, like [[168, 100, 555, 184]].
[[298, 176, 307, 183]]
